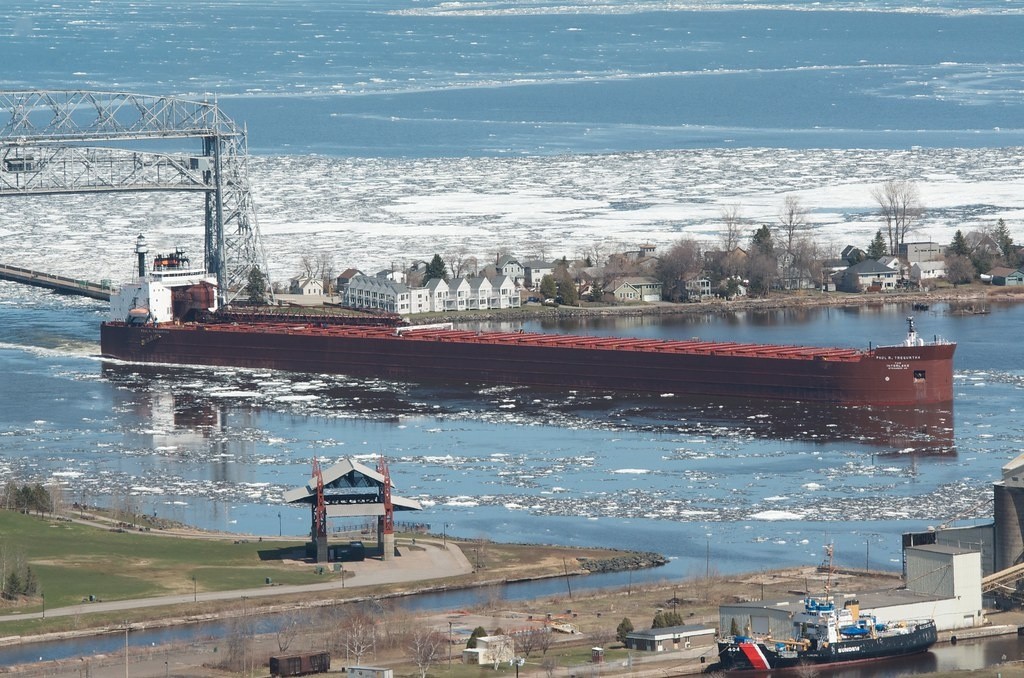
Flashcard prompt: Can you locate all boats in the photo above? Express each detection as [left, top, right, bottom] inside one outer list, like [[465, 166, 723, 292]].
[[701, 541, 938, 678], [98, 232, 960, 406]]
